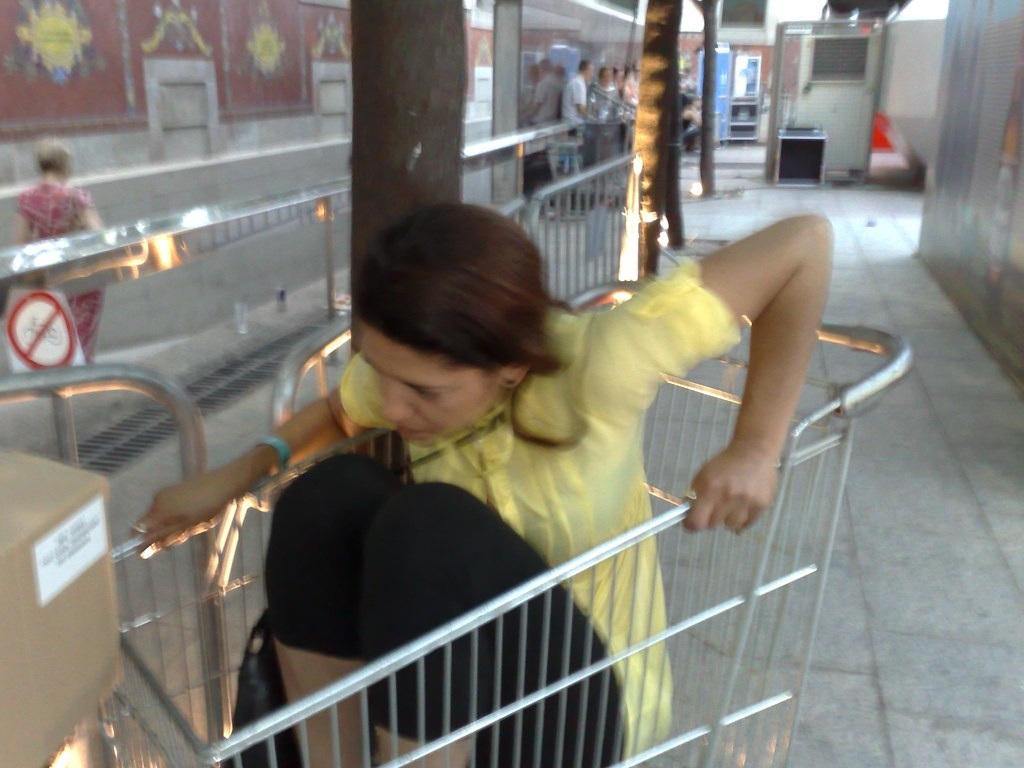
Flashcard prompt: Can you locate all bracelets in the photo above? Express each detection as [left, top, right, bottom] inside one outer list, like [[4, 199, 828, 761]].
[[257, 438, 291, 473]]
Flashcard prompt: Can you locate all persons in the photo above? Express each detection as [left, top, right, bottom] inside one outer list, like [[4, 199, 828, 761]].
[[522, 59, 565, 124], [15, 142, 108, 361], [562, 59, 701, 153], [130, 203, 833, 768]]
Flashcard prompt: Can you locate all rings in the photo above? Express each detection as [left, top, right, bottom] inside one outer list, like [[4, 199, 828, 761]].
[[135, 524, 146, 533]]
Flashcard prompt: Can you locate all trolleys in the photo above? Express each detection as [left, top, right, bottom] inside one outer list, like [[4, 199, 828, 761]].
[[78, 277, 919, 767]]
